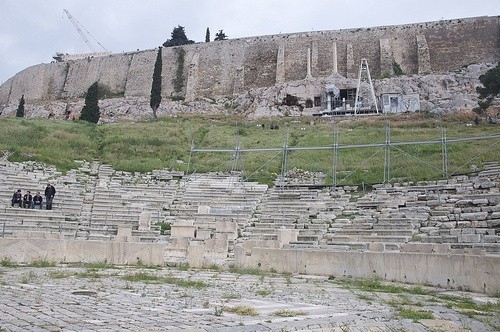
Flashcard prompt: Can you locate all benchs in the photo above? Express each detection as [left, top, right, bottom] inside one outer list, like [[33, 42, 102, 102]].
[[0, 158, 500, 252]]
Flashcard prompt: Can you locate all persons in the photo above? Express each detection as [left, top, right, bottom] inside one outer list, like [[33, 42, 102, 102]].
[[31, 192, 43, 209], [44, 183, 56, 210], [10, 189, 23, 208], [22, 191, 33, 208]]
